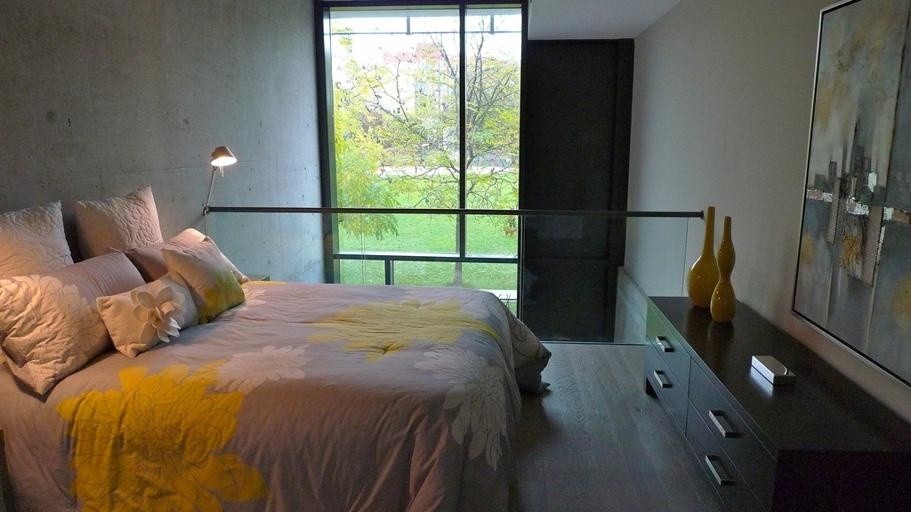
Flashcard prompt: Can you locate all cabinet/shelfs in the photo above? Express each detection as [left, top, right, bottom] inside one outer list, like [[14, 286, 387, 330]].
[[642, 294, 911, 512]]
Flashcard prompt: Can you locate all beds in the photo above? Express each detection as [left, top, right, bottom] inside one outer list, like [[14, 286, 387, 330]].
[[1, 280, 511, 510]]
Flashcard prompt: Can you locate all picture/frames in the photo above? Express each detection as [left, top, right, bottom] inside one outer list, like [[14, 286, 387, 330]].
[[790, 0, 911, 388]]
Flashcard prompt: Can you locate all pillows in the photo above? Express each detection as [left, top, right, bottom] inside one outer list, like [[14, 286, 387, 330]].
[[96, 270, 200, 358], [0, 246, 148, 395], [122, 227, 246, 285], [160, 235, 245, 324], [0, 200, 73, 276], [74, 184, 164, 260]]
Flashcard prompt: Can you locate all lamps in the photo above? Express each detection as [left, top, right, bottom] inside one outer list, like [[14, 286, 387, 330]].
[[202, 144, 239, 214]]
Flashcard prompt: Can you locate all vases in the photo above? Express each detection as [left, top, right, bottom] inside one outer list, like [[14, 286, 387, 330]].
[[685, 205, 741, 322]]
